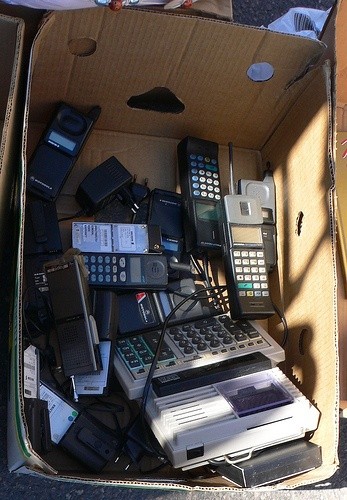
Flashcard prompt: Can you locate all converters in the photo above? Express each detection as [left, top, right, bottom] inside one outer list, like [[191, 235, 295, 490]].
[[76, 155, 140, 216], [113, 416, 167, 473]]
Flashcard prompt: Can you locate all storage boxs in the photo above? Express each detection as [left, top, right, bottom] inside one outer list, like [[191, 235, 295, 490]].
[[6, 9, 342, 491]]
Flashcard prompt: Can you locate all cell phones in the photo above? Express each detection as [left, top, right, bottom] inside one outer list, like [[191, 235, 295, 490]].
[[117, 276, 223, 338], [147, 187, 184, 277], [237, 160, 276, 273], [79, 252, 169, 291], [27, 102, 101, 204], [176, 135, 224, 283], [221, 141, 276, 321]]
